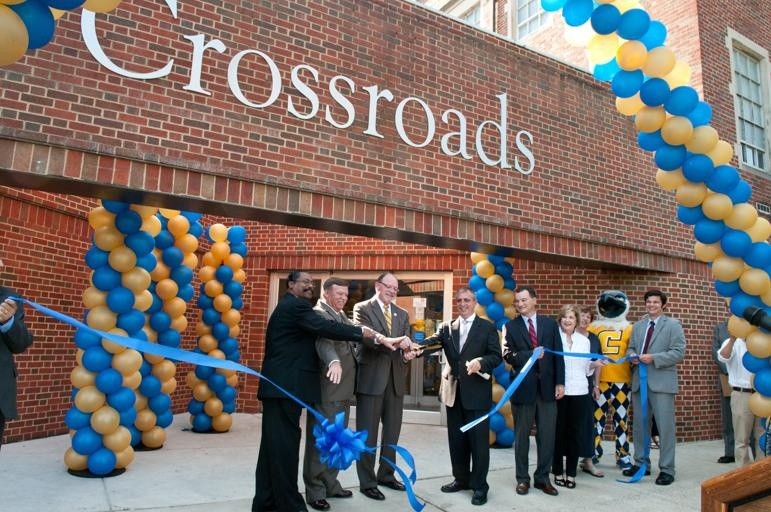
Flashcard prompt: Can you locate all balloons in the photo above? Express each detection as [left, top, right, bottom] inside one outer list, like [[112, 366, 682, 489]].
[[64, 198, 201, 476], [712, 245, 770, 455], [1, 1, 120, 69], [610, 70, 770, 265], [186, 224, 247, 432], [541, 1, 677, 80]]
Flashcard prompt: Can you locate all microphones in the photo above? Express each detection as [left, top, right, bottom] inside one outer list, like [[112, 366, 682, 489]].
[[742, 307, 769, 332]]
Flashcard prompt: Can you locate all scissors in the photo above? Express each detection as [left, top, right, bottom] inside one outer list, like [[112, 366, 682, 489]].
[[399, 341, 443, 366]]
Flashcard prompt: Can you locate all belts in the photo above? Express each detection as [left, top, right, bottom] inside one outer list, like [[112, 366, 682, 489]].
[[733, 386, 755, 393]]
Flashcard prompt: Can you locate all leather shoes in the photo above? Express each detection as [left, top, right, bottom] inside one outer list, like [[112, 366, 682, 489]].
[[361, 488, 386, 501], [441, 480, 469, 493], [334, 488, 353, 498], [533, 480, 559, 496], [622, 465, 651, 477], [566, 477, 576, 488], [718, 455, 735, 464], [379, 478, 407, 491], [554, 475, 566, 487], [515, 480, 531, 495], [310, 500, 331, 510], [470, 482, 490, 505], [655, 472, 674, 485]]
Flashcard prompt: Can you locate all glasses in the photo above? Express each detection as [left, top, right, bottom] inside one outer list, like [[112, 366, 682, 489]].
[[295, 279, 315, 286], [379, 281, 400, 293]]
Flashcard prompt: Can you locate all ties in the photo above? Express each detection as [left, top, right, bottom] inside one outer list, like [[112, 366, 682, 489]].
[[642, 320, 655, 354], [383, 304, 393, 333], [528, 319, 539, 349], [460, 321, 468, 349]]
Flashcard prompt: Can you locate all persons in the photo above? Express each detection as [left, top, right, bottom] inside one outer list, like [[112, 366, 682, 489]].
[[252, 270, 377, 512], [303, 276, 406, 511], [551, 304, 608, 489], [623, 290, 686, 485], [576, 305, 605, 478], [711, 300, 756, 464], [503, 286, 565, 495], [1, 259, 34, 451], [580, 289, 635, 470], [413, 287, 503, 506], [717, 336, 765, 469], [353, 271, 416, 500]]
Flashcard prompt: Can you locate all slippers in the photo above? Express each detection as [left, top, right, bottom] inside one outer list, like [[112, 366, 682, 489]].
[[583, 466, 605, 477]]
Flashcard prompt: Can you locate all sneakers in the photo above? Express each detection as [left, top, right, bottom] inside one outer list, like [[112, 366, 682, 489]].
[[615, 455, 633, 470]]
